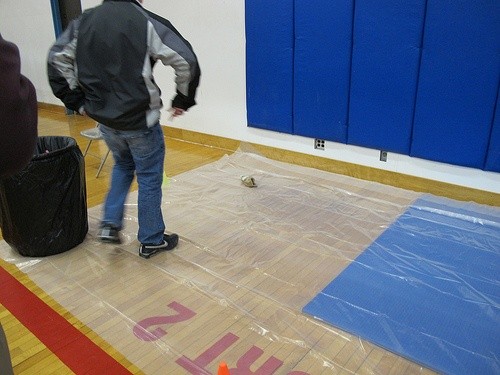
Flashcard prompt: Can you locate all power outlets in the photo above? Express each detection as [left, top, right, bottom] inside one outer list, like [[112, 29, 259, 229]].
[[380, 150, 387, 161], [314, 138, 325, 151]]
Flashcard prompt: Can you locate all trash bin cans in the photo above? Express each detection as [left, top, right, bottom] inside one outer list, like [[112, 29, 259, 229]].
[[0, 135, 90, 257]]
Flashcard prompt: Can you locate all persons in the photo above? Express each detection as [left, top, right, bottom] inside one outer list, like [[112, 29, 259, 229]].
[[46, 0, 202, 260]]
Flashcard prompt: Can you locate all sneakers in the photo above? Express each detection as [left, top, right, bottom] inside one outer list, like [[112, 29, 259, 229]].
[[97, 223, 121, 245], [138, 233, 179, 259]]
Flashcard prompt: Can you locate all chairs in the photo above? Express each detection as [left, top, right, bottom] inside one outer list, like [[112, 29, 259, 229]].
[[80, 121, 110, 179]]
